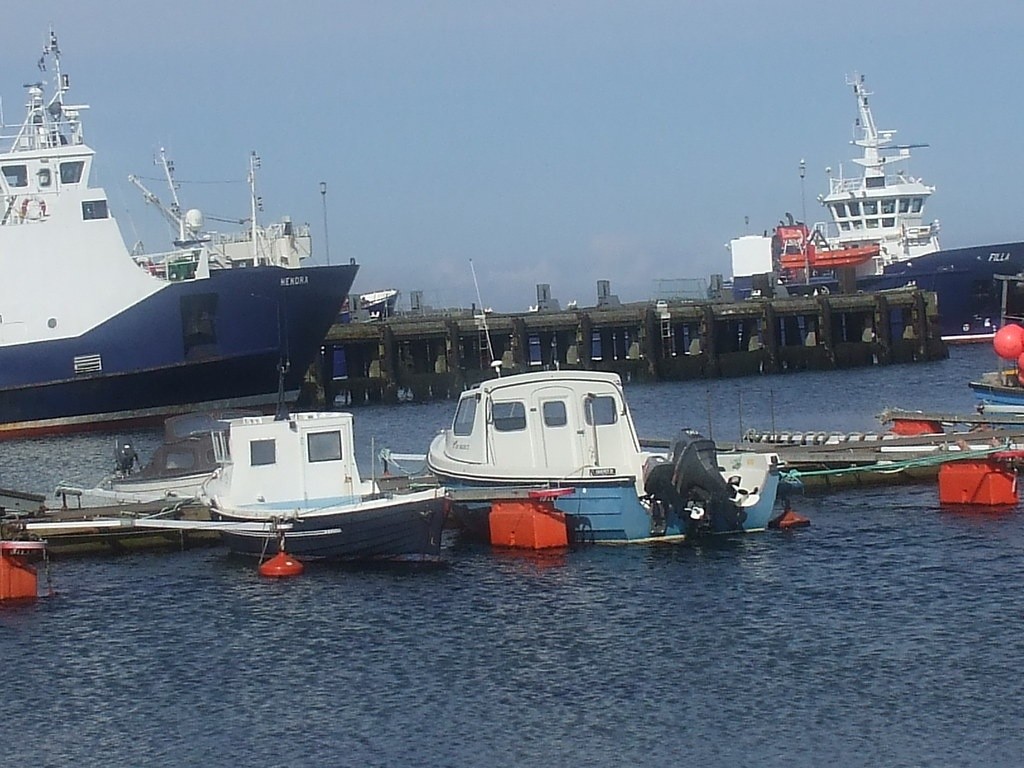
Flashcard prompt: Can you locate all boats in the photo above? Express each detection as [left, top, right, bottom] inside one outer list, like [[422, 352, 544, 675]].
[[427, 255, 788, 546], [1, 17, 361, 442], [966, 365, 1024, 407], [113, 406, 265, 486], [196, 315, 454, 563], [301, 285, 400, 410], [709, 66, 1024, 345]]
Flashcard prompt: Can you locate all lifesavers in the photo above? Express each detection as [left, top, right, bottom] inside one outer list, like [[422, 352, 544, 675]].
[[20, 195, 47, 221]]
